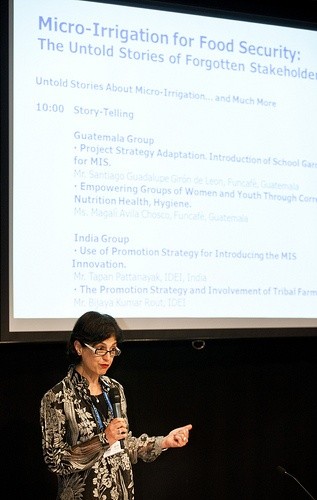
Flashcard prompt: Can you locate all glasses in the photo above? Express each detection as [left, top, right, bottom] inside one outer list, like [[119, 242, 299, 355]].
[[82, 342, 121, 357]]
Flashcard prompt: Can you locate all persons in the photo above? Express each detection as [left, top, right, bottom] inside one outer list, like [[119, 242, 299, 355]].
[[39, 311, 192, 500]]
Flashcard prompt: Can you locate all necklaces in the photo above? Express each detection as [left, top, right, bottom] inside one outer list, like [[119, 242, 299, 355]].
[[94, 395, 100, 404]]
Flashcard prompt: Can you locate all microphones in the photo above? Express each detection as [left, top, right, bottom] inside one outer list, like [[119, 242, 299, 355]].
[[279, 466, 315, 500], [109, 388, 125, 449]]
